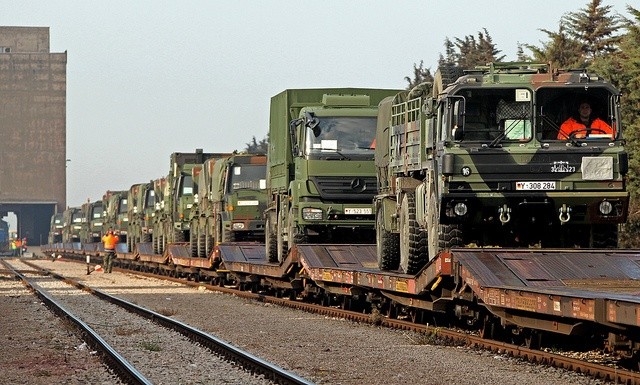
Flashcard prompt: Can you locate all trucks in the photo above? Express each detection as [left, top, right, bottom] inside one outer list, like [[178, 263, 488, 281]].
[[62, 206, 82, 244], [48, 214, 64, 244], [372, 61, 630, 274], [127, 180, 155, 252], [79, 198, 103, 244], [263, 88, 408, 263], [100, 191, 127, 244], [152, 149, 241, 254], [189, 153, 267, 257]]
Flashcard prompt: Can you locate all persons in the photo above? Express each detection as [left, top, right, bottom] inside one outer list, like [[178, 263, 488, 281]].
[[102, 227, 119, 273], [11, 237, 29, 256], [556, 101, 613, 140]]
